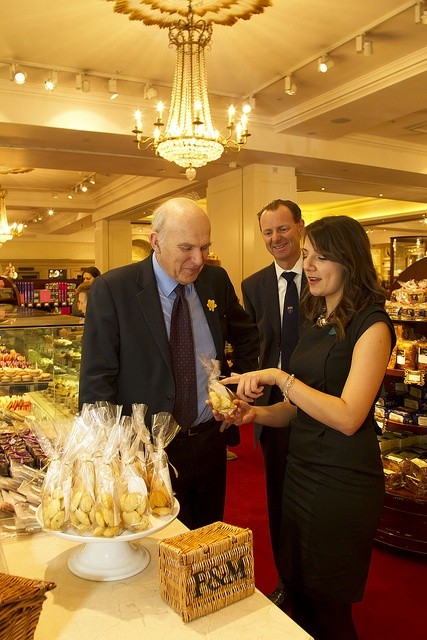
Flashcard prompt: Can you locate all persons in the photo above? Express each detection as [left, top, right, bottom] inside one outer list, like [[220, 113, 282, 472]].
[[240, 197, 327, 607], [204, 213, 396, 639], [71, 267, 100, 316], [77, 284, 90, 315], [78, 193, 260, 533]]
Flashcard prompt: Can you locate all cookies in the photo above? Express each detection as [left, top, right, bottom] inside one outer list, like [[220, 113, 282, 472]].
[[43, 454, 176, 537], [209, 391, 236, 415], [384, 278, 427, 321]]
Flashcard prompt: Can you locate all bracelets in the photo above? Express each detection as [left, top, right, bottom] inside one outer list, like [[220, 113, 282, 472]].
[[281, 372, 294, 404]]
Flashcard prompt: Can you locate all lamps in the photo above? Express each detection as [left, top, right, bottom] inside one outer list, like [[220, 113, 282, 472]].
[[9, 64, 27, 85], [0, 198, 23, 243], [284, 77, 296, 96], [107, 79, 118, 100], [131, 17, 252, 181], [79, 182, 87, 192], [413, 6, 426, 25], [241, 98, 255, 113], [355, 36, 372, 56], [43, 70, 55, 91], [142, 85, 157, 99], [76, 75, 89, 93], [88, 176, 96, 185], [317, 57, 333, 73]]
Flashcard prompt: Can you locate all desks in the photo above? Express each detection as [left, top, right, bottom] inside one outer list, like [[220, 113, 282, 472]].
[[0, 390, 313, 640]]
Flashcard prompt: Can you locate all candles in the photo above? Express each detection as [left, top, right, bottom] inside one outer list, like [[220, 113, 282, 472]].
[[133, 110, 142, 131], [152, 127, 160, 148], [153, 101, 164, 126], [225, 104, 235, 130], [236, 123, 246, 145], [132, 122, 142, 143], [192, 101, 203, 125], [239, 113, 250, 137]]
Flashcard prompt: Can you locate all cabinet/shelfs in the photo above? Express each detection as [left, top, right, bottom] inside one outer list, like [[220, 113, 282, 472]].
[[374, 257, 426, 555], [0, 304, 84, 395]]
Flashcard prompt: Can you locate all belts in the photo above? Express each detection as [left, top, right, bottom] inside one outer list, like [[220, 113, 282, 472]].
[[174, 420, 216, 438]]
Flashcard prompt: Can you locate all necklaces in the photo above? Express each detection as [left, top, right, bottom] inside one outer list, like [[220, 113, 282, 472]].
[[316, 307, 329, 329]]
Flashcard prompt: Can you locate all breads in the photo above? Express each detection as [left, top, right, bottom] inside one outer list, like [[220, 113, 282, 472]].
[[387, 323, 427, 374], [377, 431, 427, 497], [0, 336, 82, 425], [374, 380, 427, 427], [0, 422, 48, 541]]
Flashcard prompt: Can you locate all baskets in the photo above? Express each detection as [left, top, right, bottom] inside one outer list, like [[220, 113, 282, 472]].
[[158, 522, 255, 623], [0, 573, 58, 640]]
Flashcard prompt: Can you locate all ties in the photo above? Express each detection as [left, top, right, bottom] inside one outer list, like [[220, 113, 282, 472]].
[[280, 271, 302, 374], [169, 283, 198, 435]]
